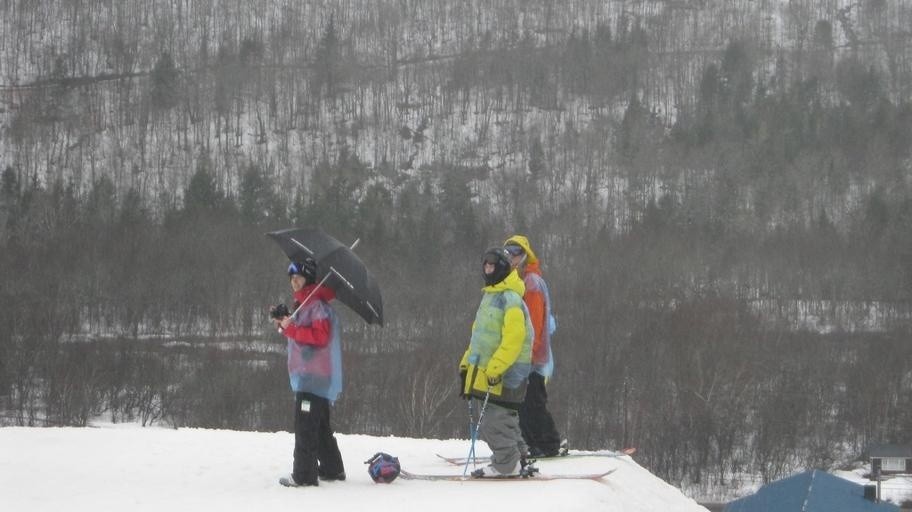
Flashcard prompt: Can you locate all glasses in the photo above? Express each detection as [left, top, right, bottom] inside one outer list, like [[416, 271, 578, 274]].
[[482, 253, 506, 266], [505, 244, 523, 256]]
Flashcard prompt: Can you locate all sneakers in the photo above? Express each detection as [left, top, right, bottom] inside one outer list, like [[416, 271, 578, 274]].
[[316, 469, 346, 482], [482, 460, 520, 478], [525, 443, 561, 457], [279, 472, 319, 488]]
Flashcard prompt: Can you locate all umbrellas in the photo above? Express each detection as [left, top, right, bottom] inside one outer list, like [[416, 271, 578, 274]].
[[265, 225, 383, 335]]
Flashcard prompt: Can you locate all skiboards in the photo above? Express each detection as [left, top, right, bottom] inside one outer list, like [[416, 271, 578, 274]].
[[436, 447, 636, 466], [398, 468, 617, 481]]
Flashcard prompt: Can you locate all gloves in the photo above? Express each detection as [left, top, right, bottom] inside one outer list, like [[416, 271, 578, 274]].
[[457, 370, 472, 400], [488, 374, 502, 387]]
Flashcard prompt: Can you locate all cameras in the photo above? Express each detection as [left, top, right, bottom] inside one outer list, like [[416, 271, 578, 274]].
[[270, 304, 290, 320]]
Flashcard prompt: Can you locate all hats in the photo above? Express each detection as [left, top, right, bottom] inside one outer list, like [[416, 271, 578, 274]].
[[286, 256, 317, 282]]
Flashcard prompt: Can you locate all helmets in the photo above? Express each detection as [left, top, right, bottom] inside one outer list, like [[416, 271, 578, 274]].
[[481, 246, 510, 284], [367, 453, 401, 484]]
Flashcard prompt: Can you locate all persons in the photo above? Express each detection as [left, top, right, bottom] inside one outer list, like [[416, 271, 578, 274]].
[[503, 234, 561, 458], [269, 259, 346, 487], [459, 244, 534, 478]]
